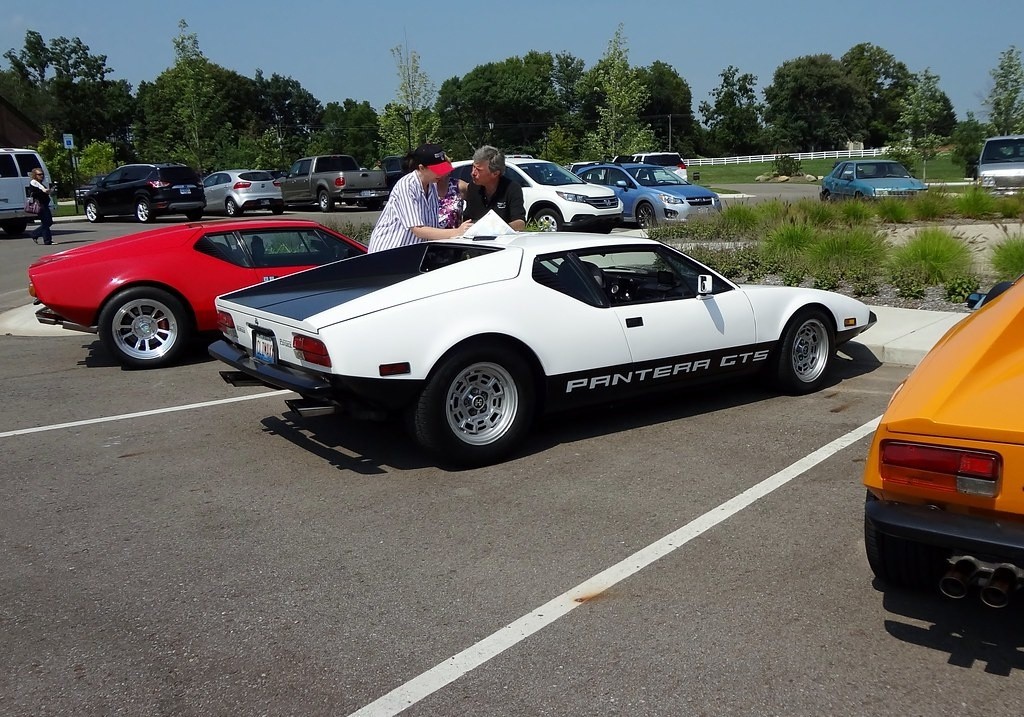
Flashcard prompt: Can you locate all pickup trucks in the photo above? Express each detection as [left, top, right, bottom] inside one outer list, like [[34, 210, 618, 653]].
[[275, 154, 391, 213]]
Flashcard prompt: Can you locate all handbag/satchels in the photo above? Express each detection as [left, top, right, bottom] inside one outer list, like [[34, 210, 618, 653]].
[[24, 192, 41, 214]]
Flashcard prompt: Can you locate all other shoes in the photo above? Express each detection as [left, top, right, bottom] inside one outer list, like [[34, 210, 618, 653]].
[[32, 238, 38, 244], [51, 242, 58, 244]]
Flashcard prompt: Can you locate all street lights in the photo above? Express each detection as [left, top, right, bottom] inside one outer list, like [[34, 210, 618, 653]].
[[111, 132, 119, 168], [544, 136, 549, 160], [279, 140, 285, 166], [487, 118, 495, 146], [404, 109, 412, 153]]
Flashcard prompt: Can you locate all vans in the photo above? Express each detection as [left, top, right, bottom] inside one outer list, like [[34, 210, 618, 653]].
[[976, 135, 1024, 194]]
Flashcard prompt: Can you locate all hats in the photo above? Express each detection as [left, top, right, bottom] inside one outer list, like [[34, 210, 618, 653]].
[[413, 144, 455, 176]]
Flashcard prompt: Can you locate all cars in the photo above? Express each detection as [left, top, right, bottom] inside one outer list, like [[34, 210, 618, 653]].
[[575, 163, 723, 229], [864, 274, 1024, 617], [817, 160, 929, 202]]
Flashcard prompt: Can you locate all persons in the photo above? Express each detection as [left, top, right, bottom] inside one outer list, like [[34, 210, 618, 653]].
[[368, 142, 527, 253], [27, 167, 57, 244]]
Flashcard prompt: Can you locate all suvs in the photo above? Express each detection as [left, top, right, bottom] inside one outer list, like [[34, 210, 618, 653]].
[[75, 173, 109, 205], [608, 152, 689, 185], [449, 153, 625, 235], [373, 155, 405, 202], [267, 168, 289, 180], [83, 163, 208, 223], [570, 161, 607, 181], [201, 169, 284, 217]]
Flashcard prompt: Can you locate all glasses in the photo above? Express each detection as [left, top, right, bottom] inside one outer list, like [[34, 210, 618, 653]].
[[36, 174, 44, 176]]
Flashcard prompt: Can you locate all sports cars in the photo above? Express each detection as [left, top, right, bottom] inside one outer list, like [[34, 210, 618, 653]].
[[0, 147, 58, 235], [206, 231, 878, 470], [26, 216, 370, 369]]
